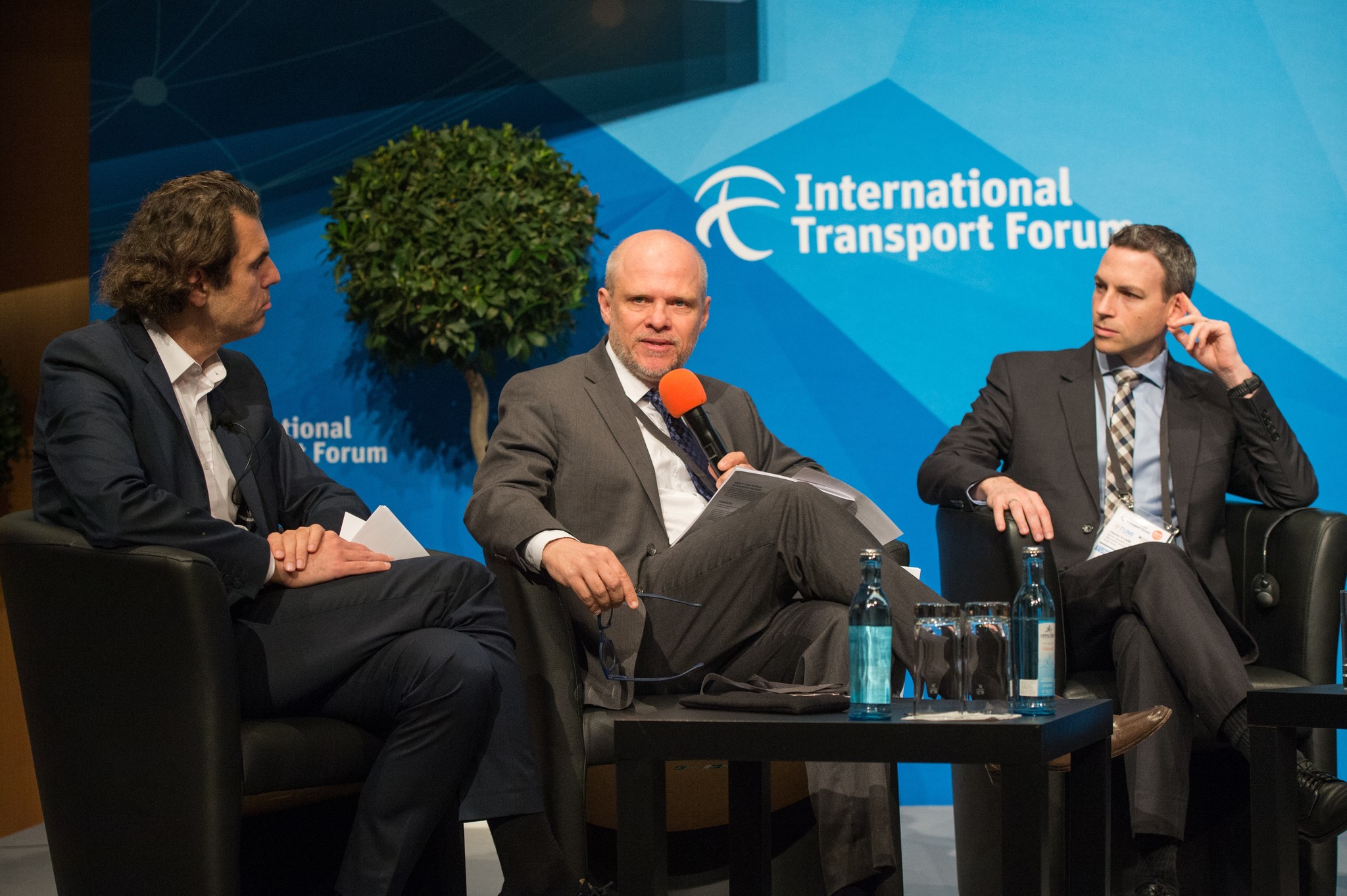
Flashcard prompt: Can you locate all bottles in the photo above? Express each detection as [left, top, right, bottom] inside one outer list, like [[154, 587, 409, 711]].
[[1011, 546, 1057, 716], [847, 548, 894, 722]]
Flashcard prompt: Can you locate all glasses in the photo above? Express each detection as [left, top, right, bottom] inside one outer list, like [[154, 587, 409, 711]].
[[598, 592, 704, 682]]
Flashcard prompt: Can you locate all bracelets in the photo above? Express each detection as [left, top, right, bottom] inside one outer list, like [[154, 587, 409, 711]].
[[1228, 375, 1261, 399]]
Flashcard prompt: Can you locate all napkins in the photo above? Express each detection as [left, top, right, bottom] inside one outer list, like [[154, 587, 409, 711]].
[[900, 710, 1022, 720]]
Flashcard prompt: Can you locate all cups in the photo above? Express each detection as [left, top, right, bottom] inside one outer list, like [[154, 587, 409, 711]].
[[913, 602, 965, 718], [963, 602, 1012, 716]]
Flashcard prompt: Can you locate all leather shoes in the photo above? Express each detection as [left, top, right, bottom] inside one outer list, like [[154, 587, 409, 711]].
[[1135, 879, 1177, 896], [574, 879, 617, 896], [984, 705, 1173, 788], [1295, 756, 1347, 844]]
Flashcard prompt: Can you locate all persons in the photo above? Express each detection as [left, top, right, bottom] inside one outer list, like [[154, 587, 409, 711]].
[[40, 169, 610, 896], [462, 229, 1173, 896], [915, 224, 1347, 896]]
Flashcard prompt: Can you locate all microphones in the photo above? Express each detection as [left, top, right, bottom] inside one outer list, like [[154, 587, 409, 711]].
[[658, 369, 729, 478], [211, 414, 230, 429]]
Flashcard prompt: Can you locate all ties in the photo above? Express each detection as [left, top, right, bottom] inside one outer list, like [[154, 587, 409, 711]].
[[1103, 369, 1143, 532], [642, 386, 716, 501]]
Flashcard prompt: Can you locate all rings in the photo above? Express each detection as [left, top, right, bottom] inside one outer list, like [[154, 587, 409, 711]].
[[1008, 499, 1020, 509]]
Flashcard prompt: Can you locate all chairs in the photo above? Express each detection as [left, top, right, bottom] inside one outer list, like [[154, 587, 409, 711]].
[[1, 508, 468, 896], [487, 544, 910, 896], [1005, 503, 1347, 896]]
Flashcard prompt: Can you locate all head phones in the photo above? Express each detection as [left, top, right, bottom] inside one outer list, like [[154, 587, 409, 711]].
[[1250, 507, 1322, 610]]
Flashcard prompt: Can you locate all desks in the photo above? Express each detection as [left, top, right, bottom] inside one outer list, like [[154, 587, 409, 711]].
[[614, 698, 1116, 896]]
